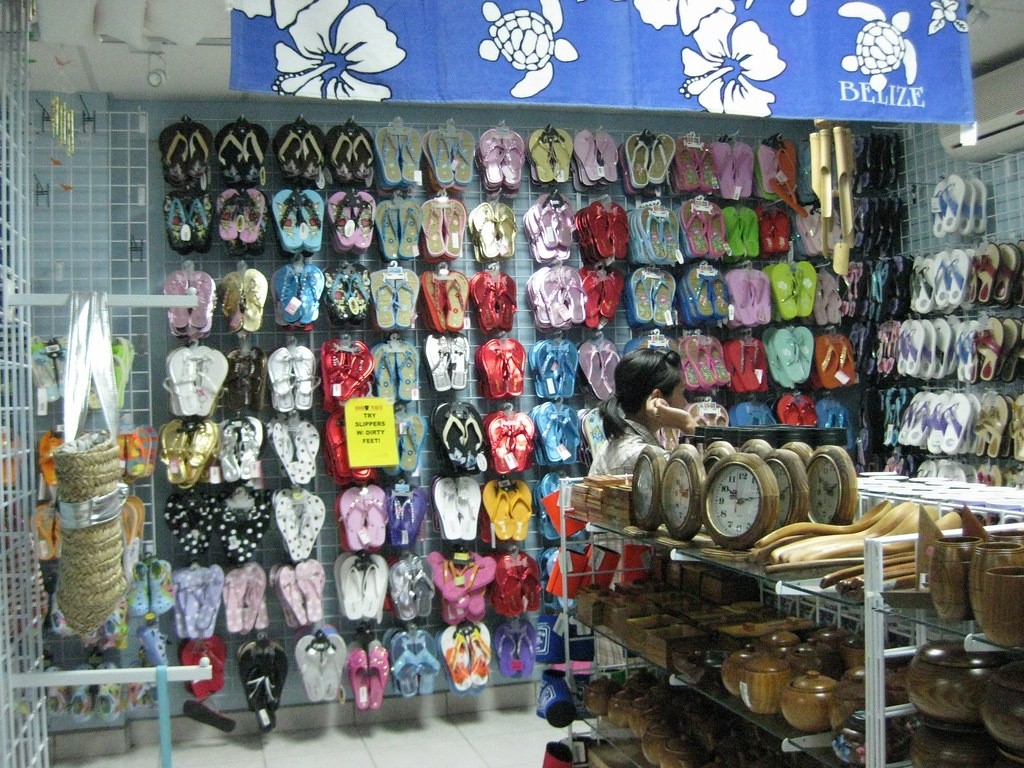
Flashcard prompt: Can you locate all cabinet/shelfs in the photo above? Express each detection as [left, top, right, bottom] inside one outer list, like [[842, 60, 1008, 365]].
[[870, 524, 1024, 768], [558, 475, 1024, 768]]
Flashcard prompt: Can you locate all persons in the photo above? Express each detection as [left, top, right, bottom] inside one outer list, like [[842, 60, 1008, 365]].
[[584, 350, 700, 729]]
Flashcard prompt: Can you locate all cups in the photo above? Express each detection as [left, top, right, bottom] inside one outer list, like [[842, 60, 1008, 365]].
[[990, 531, 1024, 544], [968, 542, 1024, 626], [930, 536, 983, 620], [983, 566, 1024, 644]]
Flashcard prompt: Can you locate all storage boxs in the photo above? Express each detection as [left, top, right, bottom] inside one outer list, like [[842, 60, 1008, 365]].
[[577, 555, 824, 673]]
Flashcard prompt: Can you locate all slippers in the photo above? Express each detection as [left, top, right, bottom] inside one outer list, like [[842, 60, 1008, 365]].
[[158, 122, 1024, 713], [30, 337, 175, 724]]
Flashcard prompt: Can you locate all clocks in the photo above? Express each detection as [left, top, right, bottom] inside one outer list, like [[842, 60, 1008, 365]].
[[631, 440, 858, 562]]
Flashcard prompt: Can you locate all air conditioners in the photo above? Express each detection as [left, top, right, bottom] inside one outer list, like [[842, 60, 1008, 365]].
[[937, 58, 1024, 165]]
